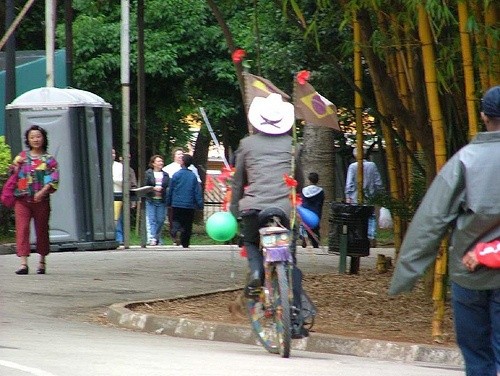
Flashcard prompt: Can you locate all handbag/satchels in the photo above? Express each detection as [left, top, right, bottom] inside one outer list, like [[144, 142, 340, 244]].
[[1, 172, 18, 209]]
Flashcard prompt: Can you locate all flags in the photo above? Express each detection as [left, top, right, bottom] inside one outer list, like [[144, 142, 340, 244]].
[[249, 73, 291, 107], [295, 78, 340, 130]]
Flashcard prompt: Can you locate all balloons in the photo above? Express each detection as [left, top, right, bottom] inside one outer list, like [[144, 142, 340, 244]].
[[206, 212, 237, 242], [297, 207, 319, 229]]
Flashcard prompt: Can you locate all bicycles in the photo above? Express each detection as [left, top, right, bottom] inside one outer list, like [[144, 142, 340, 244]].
[[237, 208, 301, 359]]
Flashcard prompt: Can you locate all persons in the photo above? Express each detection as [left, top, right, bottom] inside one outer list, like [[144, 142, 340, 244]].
[[112, 148, 137, 245], [162, 148, 204, 248], [300, 172, 325, 248], [390, 86, 500, 376], [345, 147, 387, 247], [230, 94, 309, 339], [143, 155, 169, 246], [7, 125, 60, 274]]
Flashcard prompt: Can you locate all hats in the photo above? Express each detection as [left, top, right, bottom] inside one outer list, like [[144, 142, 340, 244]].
[[481, 86, 500, 116], [247, 93, 295, 134]]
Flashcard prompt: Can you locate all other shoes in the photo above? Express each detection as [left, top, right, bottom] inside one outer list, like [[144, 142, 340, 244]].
[[37, 262, 45, 274], [243, 270, 263, 298], [16, 264, 29, 274], [292, 322, 309, 340]]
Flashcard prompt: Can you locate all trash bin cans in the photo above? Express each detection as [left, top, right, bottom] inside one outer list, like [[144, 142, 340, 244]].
[[327, 199, 375, 275]]
